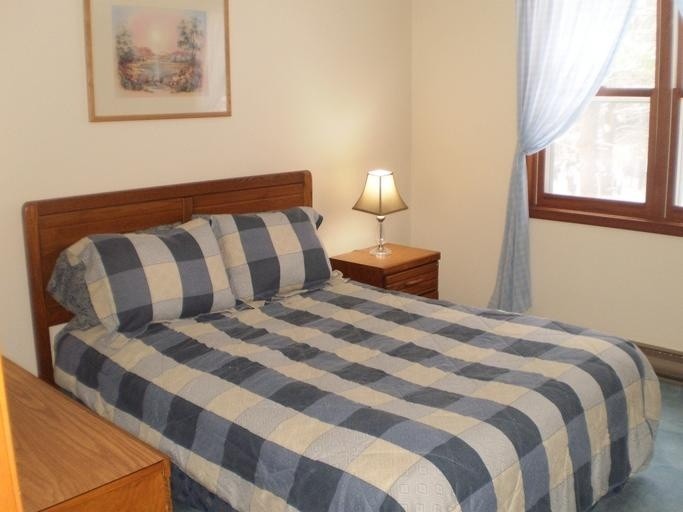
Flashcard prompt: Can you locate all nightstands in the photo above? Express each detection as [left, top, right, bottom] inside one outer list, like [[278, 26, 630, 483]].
[[328, 243, 440, 300]]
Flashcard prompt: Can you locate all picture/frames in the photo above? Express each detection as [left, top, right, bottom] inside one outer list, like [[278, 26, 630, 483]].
[[83, 0, 231, 122]]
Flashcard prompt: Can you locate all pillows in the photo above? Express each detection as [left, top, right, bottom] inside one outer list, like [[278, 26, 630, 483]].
[[48, 216, 238, 335], [202, 208, 331, 304]]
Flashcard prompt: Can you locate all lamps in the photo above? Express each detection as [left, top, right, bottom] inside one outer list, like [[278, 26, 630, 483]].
[[352, 169, 408, 257]]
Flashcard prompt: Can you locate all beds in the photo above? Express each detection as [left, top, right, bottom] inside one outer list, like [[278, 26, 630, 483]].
[[22, 171, 661, 512]]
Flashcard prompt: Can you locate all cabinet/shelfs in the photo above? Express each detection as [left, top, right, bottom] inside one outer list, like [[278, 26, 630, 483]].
[[0, 355, 172, 512]]
